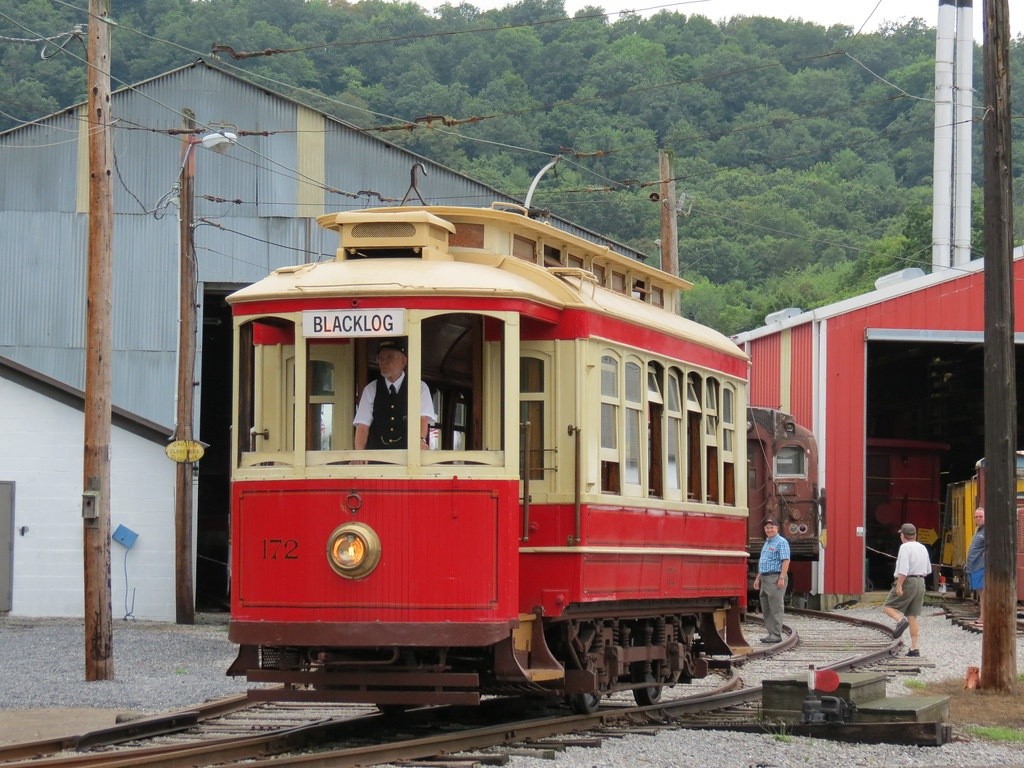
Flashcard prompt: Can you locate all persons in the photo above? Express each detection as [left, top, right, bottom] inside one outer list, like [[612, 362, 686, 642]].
[[965, 505, 988, 628], [350, 339, 438, 466], [753, 514, 792, 644], [881, 521, 933, 658]]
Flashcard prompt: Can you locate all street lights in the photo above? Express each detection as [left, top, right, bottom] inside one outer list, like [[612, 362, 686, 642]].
[[154, 132, 240, 622]]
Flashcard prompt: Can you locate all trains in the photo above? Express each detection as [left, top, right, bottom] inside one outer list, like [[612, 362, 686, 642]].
[[744, 406, 820, 605], [226, 154, 753, 714], [945, 453, 1023, 609]]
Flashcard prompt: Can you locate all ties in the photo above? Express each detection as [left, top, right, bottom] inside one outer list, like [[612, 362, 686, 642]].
[[389, 384, 397, 394]]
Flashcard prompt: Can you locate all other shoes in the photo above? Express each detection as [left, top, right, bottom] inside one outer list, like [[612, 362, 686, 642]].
[[970, 620, 984, 627], [760, 637, 782, 643], [893, 618, 909, 639], [904, 647, 920, 657]]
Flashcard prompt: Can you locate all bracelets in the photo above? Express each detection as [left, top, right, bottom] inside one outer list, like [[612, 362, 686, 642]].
[[779, 574, 787, 579], [420, 436, 427, 445]]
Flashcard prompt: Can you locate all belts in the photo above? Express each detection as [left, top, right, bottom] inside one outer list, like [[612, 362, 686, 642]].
[[896, 575, 924, 580]]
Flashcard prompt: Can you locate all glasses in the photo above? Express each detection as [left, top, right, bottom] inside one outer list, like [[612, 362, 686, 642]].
[[973, 516, 984, 519], [377, 354, 403, 361]]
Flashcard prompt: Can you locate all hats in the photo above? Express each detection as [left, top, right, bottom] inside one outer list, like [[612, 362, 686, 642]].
[[898, 523, 916, 533], [764, 517, 778, 526], [376, 340, 406, 352]]
